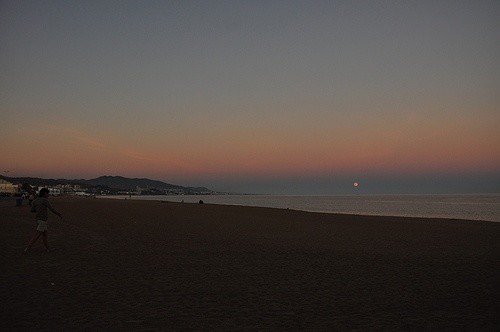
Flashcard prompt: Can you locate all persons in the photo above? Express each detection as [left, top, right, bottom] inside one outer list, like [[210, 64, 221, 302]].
[[24, 188, 64, 255], [0, 186, 186, 207]]
